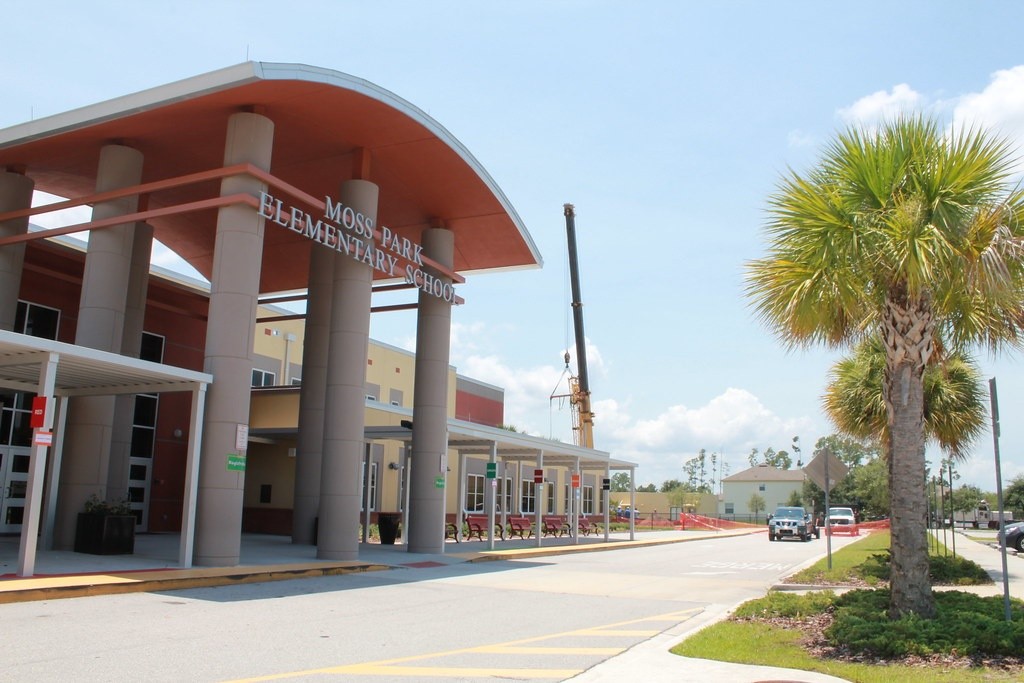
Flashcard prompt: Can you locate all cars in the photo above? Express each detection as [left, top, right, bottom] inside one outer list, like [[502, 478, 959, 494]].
[[824, 506, 855, 526], [997, 521, 1024, 553], [769, 506, 822, 543]]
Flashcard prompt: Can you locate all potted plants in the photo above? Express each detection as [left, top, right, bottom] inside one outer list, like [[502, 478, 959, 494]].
[[377, 512, 400, 545], [74, 489, 136, 556]]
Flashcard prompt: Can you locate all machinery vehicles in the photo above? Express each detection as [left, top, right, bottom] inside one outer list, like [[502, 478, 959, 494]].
[[548, 202, 637, 521]]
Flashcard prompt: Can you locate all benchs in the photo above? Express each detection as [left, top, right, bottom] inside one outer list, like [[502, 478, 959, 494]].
[[445, 523, 459, 544], [467, 516, 505, 542], [542, 518, 572, 539], [578, 519, 598, 536], [509, 518, 535, 540]]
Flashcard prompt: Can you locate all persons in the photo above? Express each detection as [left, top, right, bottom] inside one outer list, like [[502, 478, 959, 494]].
[[634, 507, 640, 519], [609, 506, 630, 518]]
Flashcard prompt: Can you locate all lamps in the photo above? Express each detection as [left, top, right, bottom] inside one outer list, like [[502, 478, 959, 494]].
[[174, 429, 182, 437], [389, 462, 400, 470]]
